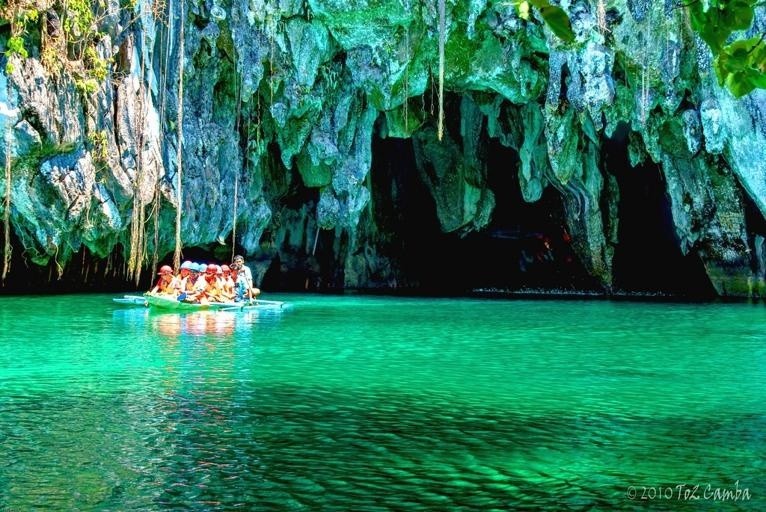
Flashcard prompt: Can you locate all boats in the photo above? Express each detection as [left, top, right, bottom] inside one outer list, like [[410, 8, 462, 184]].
[[140, 291, 258, 312]]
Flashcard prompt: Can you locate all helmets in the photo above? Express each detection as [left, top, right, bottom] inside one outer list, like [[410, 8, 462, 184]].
[[157, 265, 173, 275], [179, 261, 231, 275]]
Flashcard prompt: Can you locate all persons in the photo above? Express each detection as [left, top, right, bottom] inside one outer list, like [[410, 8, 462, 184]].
[[148, 255, 261, 306]]
